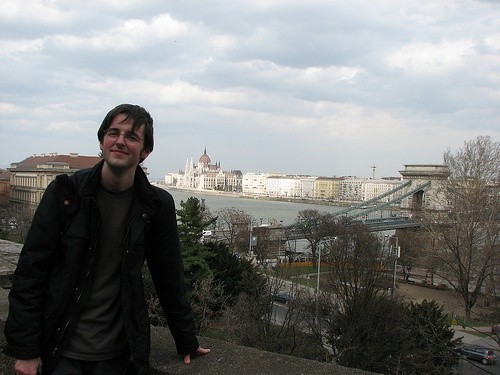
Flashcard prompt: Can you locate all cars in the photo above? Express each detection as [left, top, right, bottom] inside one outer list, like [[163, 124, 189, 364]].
[[455, 345, 498, 365]]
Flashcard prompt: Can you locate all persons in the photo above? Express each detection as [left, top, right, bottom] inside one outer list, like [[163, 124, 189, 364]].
[[2, 102, 212, 375]]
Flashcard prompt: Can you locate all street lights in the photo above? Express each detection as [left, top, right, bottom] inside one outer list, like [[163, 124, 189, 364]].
[[313, 236, 337, 329], [384, 234, 401, 303]]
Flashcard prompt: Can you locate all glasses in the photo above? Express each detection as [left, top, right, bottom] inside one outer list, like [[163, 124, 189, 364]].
[[103, 129, 145, 143]]
